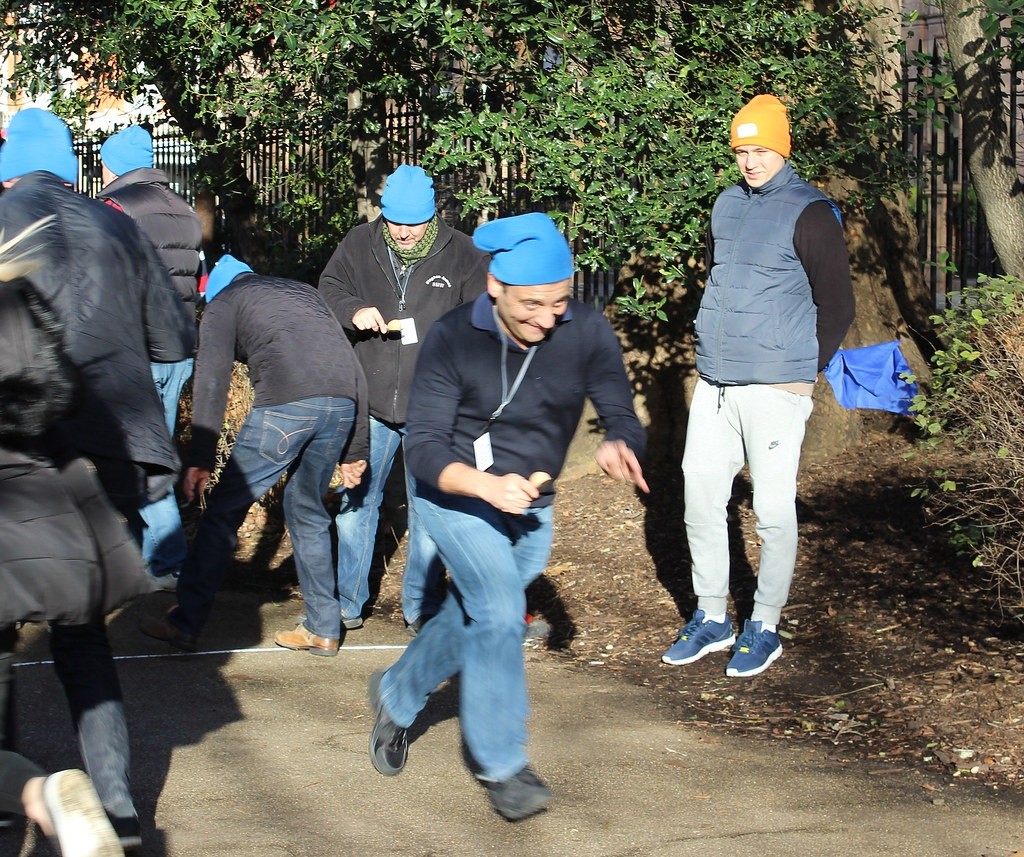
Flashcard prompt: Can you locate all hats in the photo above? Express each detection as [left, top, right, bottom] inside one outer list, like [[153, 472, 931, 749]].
[[0, 108, 79, 184], [204, 254, 254, 303], [381, 165, 436, 225], [100, 125, 154, 177], [473, 212, 574, 286], [730, 94, 792, 158]]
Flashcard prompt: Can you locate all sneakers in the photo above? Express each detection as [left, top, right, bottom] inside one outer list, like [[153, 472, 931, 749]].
[[108, 813, 144, 850], [367, 670, 408, 776], [726, 619, 783, 677], [479, 764, 551, 820], [37, 768, 125, 856], [662, 609, 736, 666], [145, 567, 180, 592]]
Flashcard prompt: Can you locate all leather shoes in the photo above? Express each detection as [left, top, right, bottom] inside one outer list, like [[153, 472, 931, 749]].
[[274, 623, 339, 656], [137, 613, 198, 652]]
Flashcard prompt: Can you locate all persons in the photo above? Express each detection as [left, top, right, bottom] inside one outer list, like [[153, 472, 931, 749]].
[[317, 165, 495, 639], [90, 125, 208, 594], [2, 214, 180, 857], [363, 213, 651, 822], [0, 103, 193, 849], [659, 91, 859, 678], [139, 253, 370, 657]]
